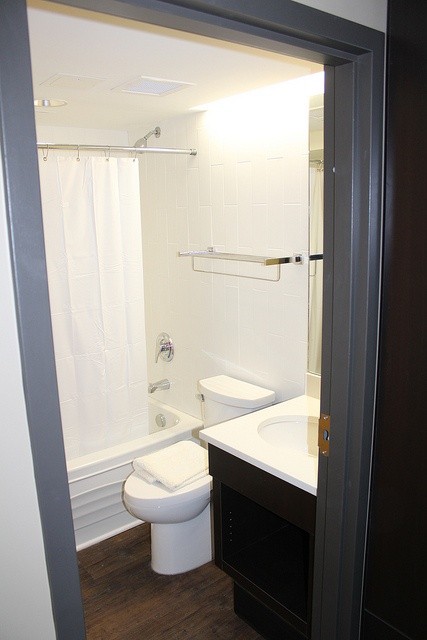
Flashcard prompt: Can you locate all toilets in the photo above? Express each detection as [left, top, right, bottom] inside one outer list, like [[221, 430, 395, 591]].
[[123, 374, 275, 576]]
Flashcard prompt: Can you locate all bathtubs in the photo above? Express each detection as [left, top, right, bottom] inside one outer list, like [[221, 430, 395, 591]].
[[60, 394, 203, 553]]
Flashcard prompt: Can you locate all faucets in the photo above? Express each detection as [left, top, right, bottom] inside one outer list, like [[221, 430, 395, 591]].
[[147, 379, 170, 394]]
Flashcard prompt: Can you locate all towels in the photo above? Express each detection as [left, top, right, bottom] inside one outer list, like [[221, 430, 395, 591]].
[[133, 438, 209, 490]]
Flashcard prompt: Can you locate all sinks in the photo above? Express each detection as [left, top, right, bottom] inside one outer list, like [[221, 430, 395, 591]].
[[256, 414, 323, 462]]
